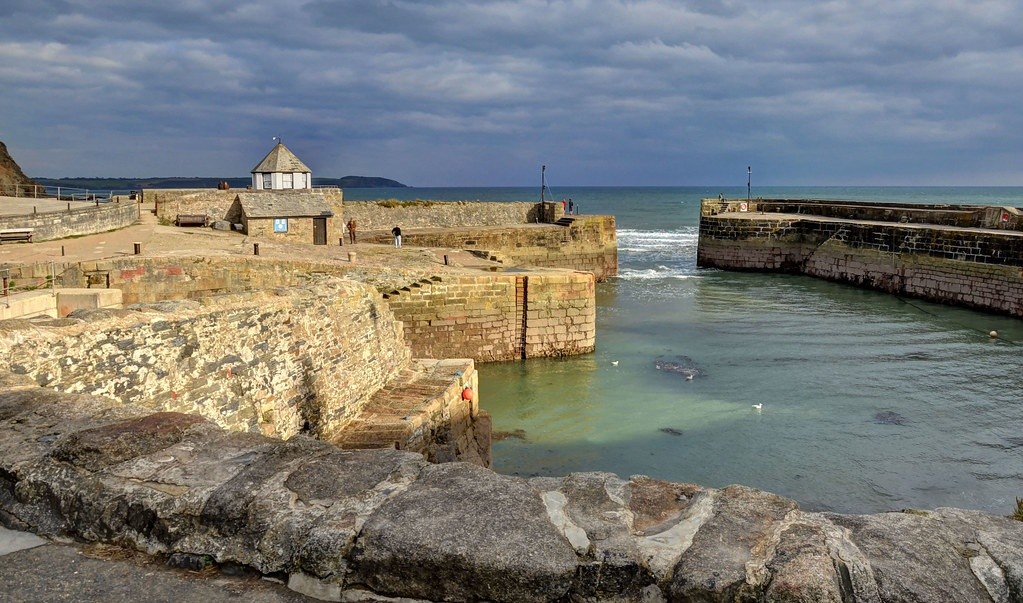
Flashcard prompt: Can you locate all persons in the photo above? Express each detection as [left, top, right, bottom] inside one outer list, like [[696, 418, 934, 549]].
[[391, 225, 402, 248], [347, 218, 357, 244], [562, 199, 567, 213], [569, 199, 573, 215]]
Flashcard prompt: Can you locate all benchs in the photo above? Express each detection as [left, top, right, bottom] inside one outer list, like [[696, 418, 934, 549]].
[[0, 231, 33, 243], [176, 214, 209, 227]]
